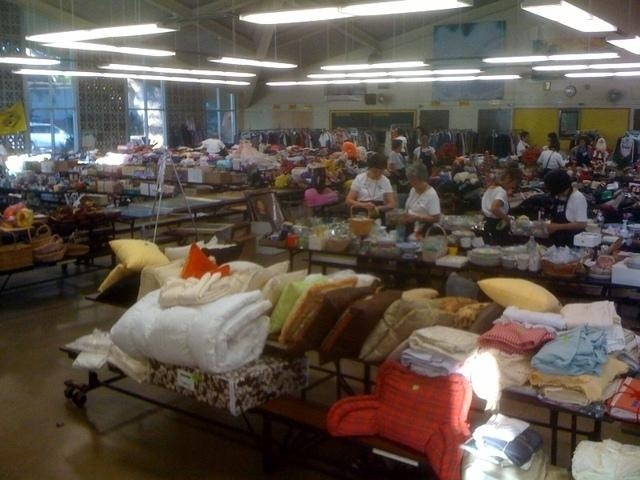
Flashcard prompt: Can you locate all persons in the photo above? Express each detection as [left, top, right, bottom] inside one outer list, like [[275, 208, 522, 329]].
[[345, 128, 594, 249], [197, 138, 229, 156]]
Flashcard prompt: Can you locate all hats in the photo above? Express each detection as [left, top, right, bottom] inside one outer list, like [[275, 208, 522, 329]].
[[543, 170, 577, 189]]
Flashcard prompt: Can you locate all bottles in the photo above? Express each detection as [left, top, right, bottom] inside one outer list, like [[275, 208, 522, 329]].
[[270, 204, 639, 288]]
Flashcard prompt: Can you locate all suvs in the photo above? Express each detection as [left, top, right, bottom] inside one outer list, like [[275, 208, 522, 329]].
[[29, 122, 72, 150]]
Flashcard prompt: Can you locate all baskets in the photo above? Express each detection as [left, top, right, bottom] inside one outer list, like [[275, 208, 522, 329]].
[[32, 224, 67, 263], [421, 223, 448, 262], [349, 205, 372, 237]]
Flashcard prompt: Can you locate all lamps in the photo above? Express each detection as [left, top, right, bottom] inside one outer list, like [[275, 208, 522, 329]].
[[0, 0, 640, 88]]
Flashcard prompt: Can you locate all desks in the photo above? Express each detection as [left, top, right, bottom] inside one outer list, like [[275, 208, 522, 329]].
[[61, 319, 442, 479], [3, 126, 640, 311]]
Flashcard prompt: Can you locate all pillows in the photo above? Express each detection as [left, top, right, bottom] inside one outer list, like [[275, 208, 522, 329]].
[[277, 276, 358, 346], [288, 280, 380, 351], [359, 287, 439, 354], [313, 288, 400, 355], [267, 268, 351, 335], [364, 304, 489, 363], [391, 295, 478, 326], [319, 360, 473, 479]]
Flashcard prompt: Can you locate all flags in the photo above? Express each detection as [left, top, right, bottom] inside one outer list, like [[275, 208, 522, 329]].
[[0, 96, 28, 138]]
